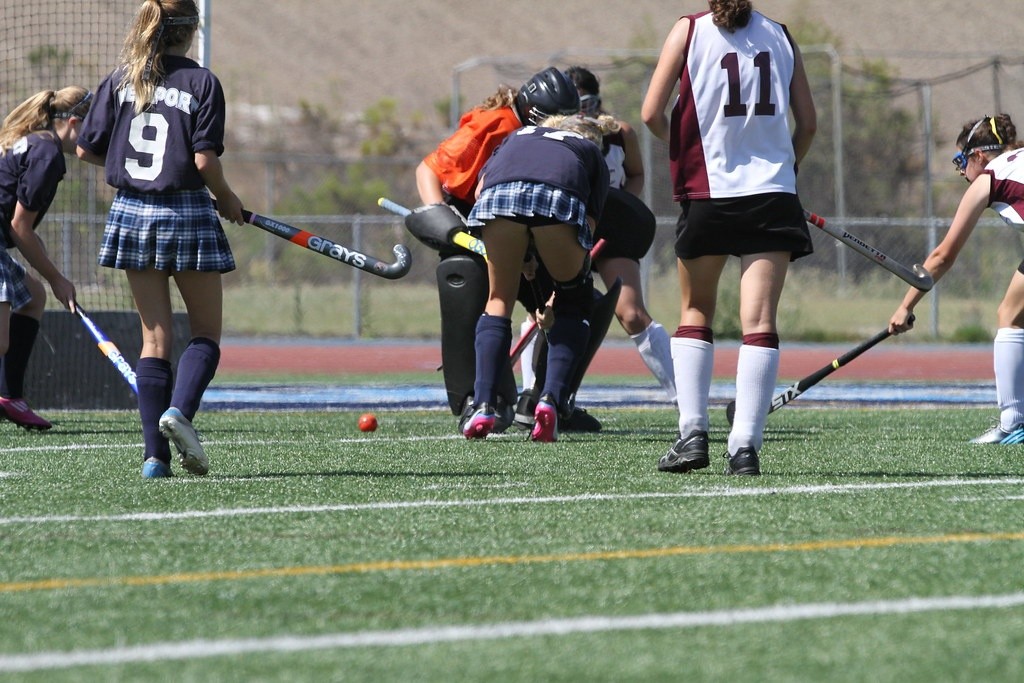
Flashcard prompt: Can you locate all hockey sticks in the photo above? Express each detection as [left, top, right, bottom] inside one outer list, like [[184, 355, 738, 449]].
[[725, 313, 916, 426], [73, 299, 138, 398], [508, 239, 605, 368], [209, 196, 413, 279], [800, 207, 935, 293], [377, 195, 488, 258]]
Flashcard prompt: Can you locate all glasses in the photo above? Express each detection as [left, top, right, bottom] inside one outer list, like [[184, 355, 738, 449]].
[[952, 153, 968, 170], [578, 95, 601, 114]]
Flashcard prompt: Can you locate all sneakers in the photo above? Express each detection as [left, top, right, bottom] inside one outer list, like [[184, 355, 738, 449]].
[[515, 388, 602, 432], [524, 391, 557, 443], [463, 402, 495, 438], [159, 407, 209, 475], [659, 430, 710, 475], [0, 397, 52, 433], [142, 456, 175, 479], [969, 423, 1024, 445], [722, 446, 761, 476], [458, 395, 515, 431]]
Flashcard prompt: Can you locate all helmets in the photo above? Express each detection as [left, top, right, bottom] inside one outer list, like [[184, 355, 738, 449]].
[[515, 67, 581, 125]]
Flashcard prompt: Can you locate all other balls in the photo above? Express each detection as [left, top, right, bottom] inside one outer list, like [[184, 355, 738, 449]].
[[359, 413, 378, 431]]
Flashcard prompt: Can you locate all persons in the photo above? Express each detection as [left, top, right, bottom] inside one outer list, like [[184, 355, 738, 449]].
[[888, 114, 1024, 445], [416, 67, 678, 444], [0, 86, 95, 430], [641, 0, 816, 477], [73, 0, 244, 478]]
